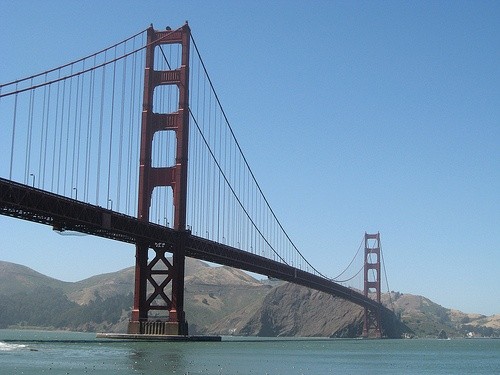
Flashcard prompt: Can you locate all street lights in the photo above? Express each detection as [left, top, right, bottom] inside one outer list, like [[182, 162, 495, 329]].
[[30, 173, 37, 188], [73, 187, 80, 200], [108, 198, 113, 211], [164, 217, 364, 298]]
